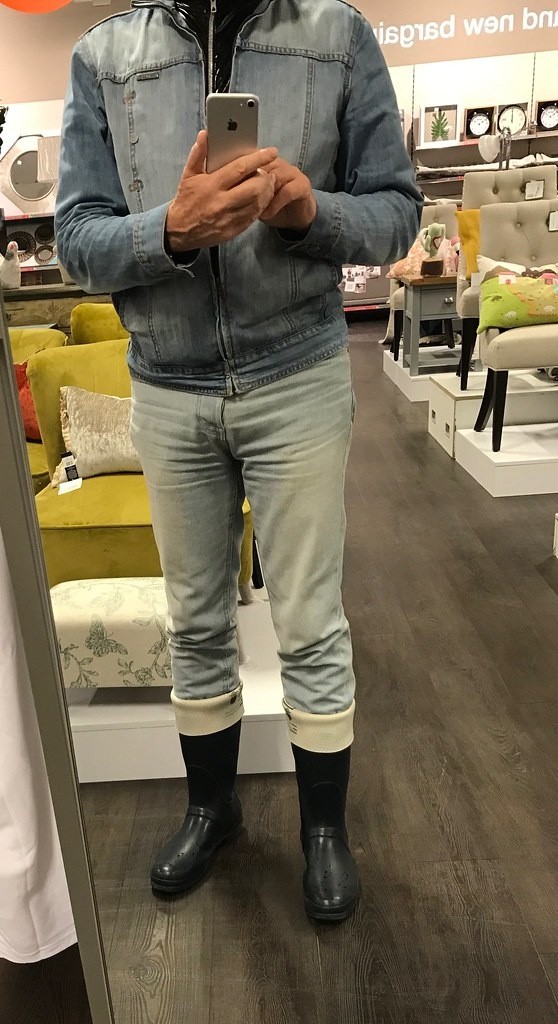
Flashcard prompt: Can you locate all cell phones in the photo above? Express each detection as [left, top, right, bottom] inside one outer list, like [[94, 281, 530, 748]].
[[204, 92, 259, 178]]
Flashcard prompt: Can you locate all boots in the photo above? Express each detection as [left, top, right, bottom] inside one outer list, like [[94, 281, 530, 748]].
[[150, 679, 244, 893], [277, 697, 361, 920]]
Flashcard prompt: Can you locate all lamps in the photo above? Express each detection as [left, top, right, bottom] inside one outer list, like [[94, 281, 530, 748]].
[[478, 127, 512, 170], [37, 135, 80, 284]]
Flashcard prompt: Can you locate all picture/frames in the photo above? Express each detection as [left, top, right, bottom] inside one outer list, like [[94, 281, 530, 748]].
[[419, 99, 461, 149]]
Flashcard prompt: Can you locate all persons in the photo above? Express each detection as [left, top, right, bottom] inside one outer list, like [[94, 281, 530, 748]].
[[54, 0, 424, 921]]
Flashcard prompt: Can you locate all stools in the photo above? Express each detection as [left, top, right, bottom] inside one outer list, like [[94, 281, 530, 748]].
[[48, 578, 177, 690]]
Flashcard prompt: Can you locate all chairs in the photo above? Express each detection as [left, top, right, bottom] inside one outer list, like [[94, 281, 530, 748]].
[[10, 303, 263, 667], [378, 165, 558, 452]]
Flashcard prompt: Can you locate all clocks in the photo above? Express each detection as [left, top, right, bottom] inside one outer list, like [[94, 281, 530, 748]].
[[533, 100, 558, 132], [495, 102, 532, 138], [464, 105, 499, 143]]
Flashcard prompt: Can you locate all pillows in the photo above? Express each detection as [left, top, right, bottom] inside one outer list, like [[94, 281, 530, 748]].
[[385, 227, 459, 280], [453, 208, 482, 280], [474, 255, 558, 334], [51, 385, 144, 484], [12, 347, 48, 443]]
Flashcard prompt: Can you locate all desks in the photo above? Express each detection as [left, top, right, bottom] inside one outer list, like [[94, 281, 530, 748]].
[[401, 274, 484, 376]]
